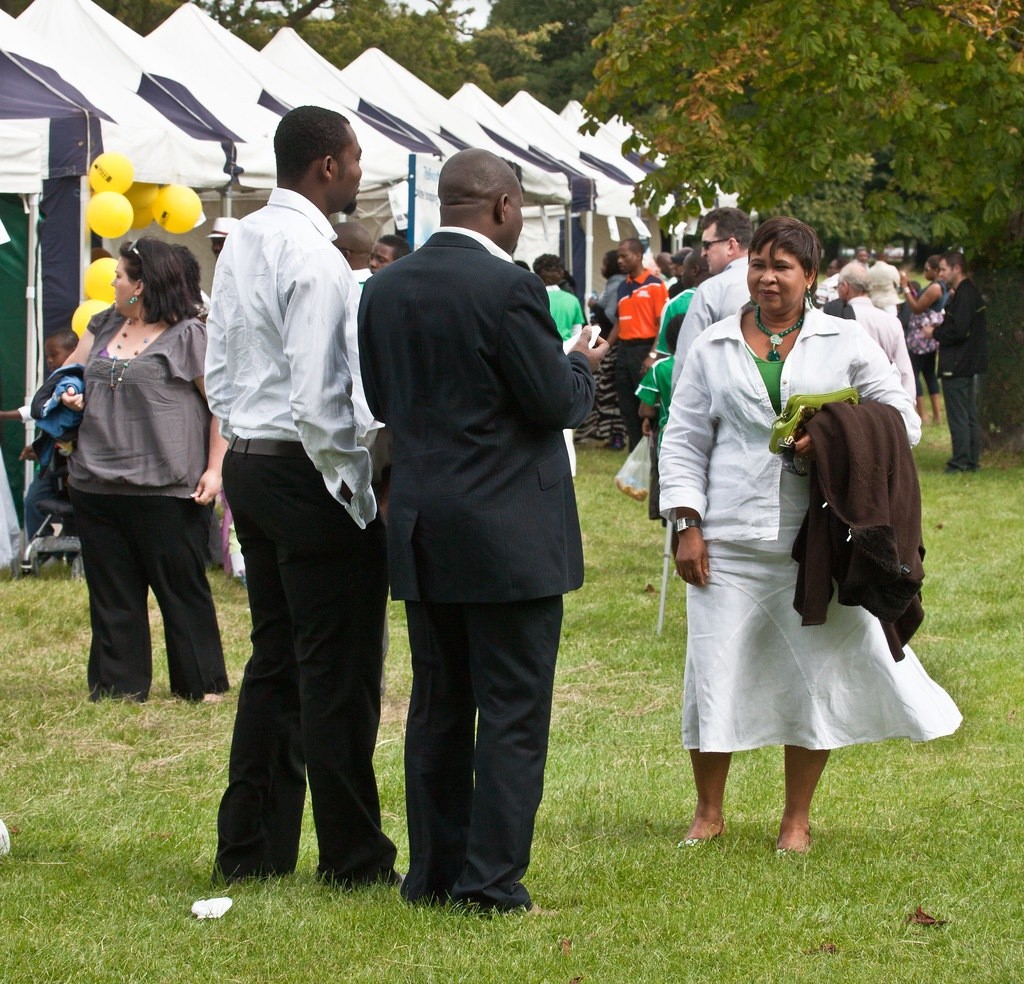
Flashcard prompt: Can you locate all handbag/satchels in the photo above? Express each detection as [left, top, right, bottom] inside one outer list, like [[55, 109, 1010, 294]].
[[769, 387, 858, 455], [615, 430, 654, 502]]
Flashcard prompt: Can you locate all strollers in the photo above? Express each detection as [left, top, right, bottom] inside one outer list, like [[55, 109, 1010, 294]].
[[20, 466, 84, 576]]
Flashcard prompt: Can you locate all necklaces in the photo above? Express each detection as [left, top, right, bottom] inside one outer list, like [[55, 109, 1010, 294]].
[[754, 304, 804, 361], [109, 317, 166, 389]]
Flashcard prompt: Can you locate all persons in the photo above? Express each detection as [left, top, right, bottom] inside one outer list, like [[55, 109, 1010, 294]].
[[922, 252, 985, 473], [816, 259, 918, 410], [206, 103, 396, 891], [358, 146, 609, 907], [0, 104, 990, 580], [602, 239, 670, 459], [660, 215, 966, 868], [34, 237, 232, 707], [671, 206, 755, 399]]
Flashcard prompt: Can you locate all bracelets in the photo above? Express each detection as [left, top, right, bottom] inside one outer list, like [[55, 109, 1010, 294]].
[[903, 287, 911, 294], [649, 352, 658, 359]]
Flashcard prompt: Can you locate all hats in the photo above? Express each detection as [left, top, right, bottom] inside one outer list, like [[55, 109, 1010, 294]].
[[204, 216, 240, 239]]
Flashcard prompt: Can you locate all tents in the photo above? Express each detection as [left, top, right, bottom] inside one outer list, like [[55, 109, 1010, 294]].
[[0, 12, 109, 596], [18, 0, 750, 322]]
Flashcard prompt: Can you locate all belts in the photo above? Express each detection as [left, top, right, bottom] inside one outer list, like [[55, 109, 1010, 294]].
[[620, 337, 657, 347], [228, 436, 307, 459]]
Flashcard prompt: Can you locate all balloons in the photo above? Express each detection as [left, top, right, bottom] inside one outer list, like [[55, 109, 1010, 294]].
[[71, 301, 113, 341], [154, 185, 202, 235], [125, 181, 158, 229], [85, 192, 133, 239], [89, 154, 134, 195], [85, 257, 120, 303]]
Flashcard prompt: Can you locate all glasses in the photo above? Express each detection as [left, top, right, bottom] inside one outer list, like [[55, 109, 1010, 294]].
[[833, 285, 842, 291], [700, 235, 741, 251], [781, 445, 809, 478], [128, 236, 144, 263]]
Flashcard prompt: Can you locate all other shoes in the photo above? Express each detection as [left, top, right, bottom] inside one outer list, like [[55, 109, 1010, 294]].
[[676, 817, 725, 849], [602, 440, 625, 452], [775, 825, 815, 857], [944, 464, 962, 474]]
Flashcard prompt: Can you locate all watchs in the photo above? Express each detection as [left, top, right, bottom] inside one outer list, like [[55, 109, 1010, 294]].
[[675, 516, 701, 533]]
